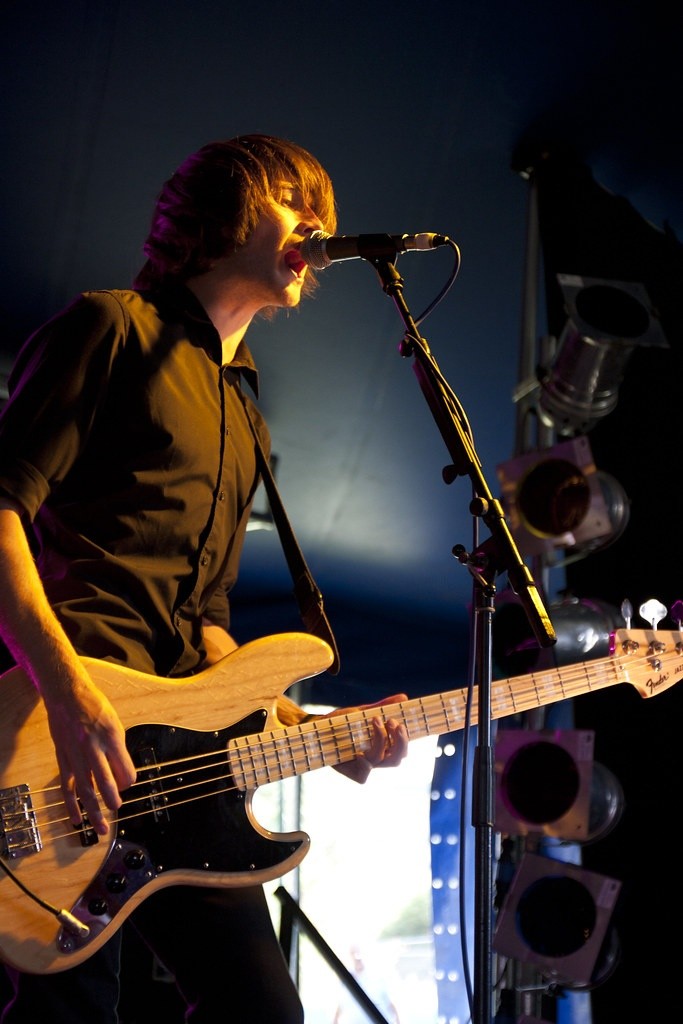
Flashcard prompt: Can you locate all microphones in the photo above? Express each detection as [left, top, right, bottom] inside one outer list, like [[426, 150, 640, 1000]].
[[301, 230, 450, 270]]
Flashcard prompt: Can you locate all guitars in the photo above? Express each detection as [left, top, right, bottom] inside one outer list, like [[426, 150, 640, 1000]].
[[0, 597, 683, 975]]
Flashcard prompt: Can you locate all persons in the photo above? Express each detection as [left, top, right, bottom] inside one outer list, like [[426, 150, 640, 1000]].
[[0, 133, 410, 1023]]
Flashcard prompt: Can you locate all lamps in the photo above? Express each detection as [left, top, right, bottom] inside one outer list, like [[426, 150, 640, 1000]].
[[491, 271, 652, 991]]
